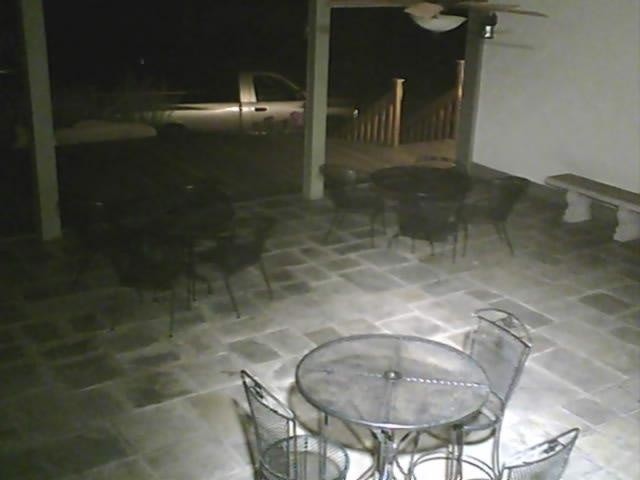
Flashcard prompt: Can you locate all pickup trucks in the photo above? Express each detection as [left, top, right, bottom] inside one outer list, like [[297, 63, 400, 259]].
[[112, 67, 360, 143]]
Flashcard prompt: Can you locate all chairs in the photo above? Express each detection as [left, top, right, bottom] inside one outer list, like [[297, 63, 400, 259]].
[[70, 165, 274, 340], [318, 157, 530, 264], [403, 308, 534, 478], [497, 426, 580, 479], [239, 368, 351, 479]]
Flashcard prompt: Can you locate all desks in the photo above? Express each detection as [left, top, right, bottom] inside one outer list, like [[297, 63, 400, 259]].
[[293, 333, 493, 479]]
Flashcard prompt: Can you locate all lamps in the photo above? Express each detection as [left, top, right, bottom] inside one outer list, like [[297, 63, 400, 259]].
[[403, 1, 443, 18], [408, 13, 467, 33]]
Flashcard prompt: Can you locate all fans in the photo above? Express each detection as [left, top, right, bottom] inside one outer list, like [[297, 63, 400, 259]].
[[328, 0, 546, 18]]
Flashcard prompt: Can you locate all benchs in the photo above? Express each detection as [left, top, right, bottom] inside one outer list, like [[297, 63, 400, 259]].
[[545, 173, 640, 242]]
